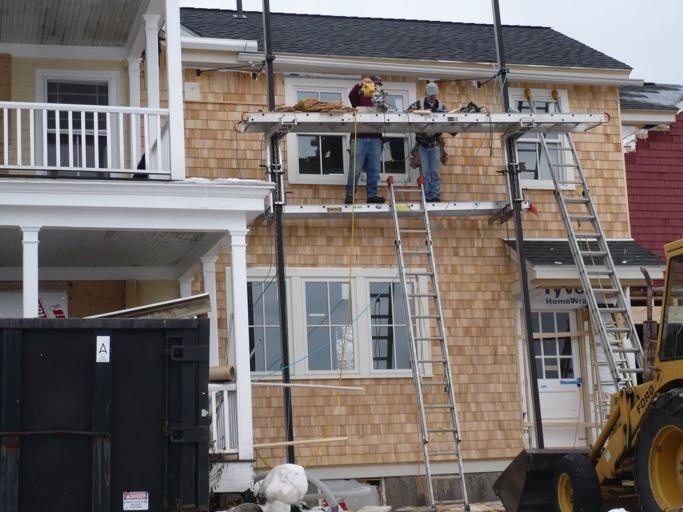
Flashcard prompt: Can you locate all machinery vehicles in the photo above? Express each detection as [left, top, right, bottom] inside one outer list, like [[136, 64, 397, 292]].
[[491, 239, 682, 511]]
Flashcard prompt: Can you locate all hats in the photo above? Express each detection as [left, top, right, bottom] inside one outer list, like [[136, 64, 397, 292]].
[[368, 75, 384, 86], [423, 82, 439, 96]]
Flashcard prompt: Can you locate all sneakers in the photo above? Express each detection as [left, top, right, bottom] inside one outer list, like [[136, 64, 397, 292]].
[[367, 196, 386, 205], [343, 195, 354, 204], [422, 196, 441, 204]]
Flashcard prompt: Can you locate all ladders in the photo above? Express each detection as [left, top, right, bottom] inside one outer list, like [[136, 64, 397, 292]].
[[587, 304, 635, 449], [387, 176, 472, 512], [525, 88, 645, 391]]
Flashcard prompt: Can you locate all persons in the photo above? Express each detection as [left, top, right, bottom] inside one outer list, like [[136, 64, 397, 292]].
[[407, 83, 449, 202], [345, 72, 389, 203], [230, 464, 309, 512]]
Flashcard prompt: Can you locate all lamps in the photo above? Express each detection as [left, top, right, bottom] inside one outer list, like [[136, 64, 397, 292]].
[[477, 67, 509, 89], [252, 55, 276, 80]]
